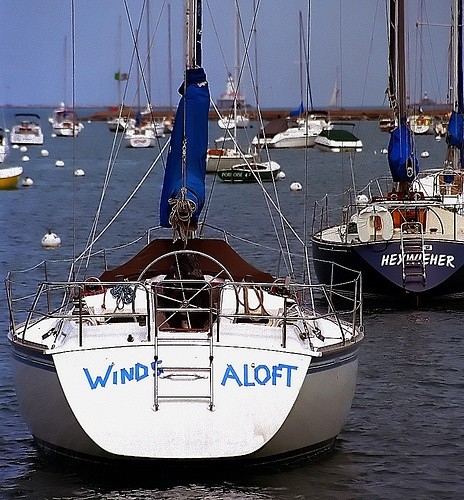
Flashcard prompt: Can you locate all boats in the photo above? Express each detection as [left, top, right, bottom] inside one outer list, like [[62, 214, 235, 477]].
[[0, 126, 9, 163], [48, 103, 82, 137], [0, 166, 24, 189], [108, 0, 464, 209], [9, 112, 44, 146]]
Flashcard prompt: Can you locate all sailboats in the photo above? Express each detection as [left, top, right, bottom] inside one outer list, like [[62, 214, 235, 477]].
[[309, 0, 464, 308], [5, 0, 365, 485]]
